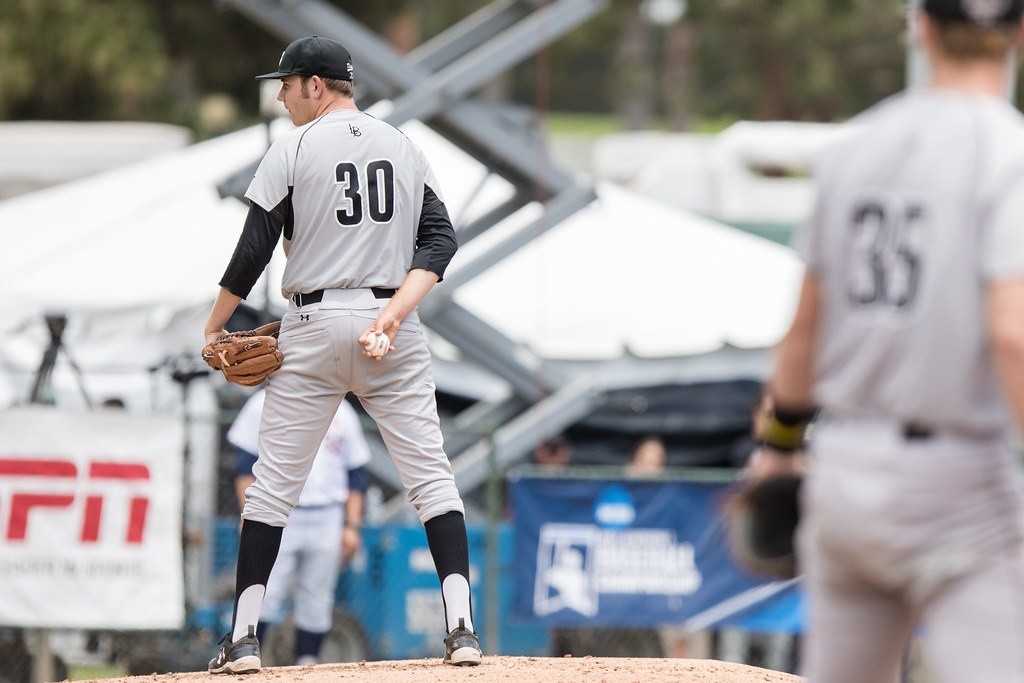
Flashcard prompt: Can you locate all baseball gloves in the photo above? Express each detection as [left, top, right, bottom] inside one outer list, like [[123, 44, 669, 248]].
[[718, 469, 803, 582], [200, 320, 285, 388]]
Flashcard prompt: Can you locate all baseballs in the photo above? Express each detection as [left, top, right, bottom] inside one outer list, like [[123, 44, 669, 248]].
[[364, 331, 391, 357]]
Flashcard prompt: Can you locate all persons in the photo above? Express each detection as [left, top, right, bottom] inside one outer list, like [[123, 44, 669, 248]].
[[204, 34, 482, 675], [726, 0, 1024, 683], [227, 384, 369, 666]]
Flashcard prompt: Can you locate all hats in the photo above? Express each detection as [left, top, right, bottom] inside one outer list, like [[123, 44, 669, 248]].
[[255, 35, 354, 80], [922, 0, 1024, 32]]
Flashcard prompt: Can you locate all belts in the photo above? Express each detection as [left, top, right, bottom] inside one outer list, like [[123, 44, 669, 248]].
[[898, 426, 938, 444], [295, 287, 394, 306]]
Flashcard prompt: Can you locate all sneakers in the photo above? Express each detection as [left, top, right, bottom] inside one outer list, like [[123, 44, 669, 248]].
[[208, 624, 260, 672], [443, 619, 482, 664]]
